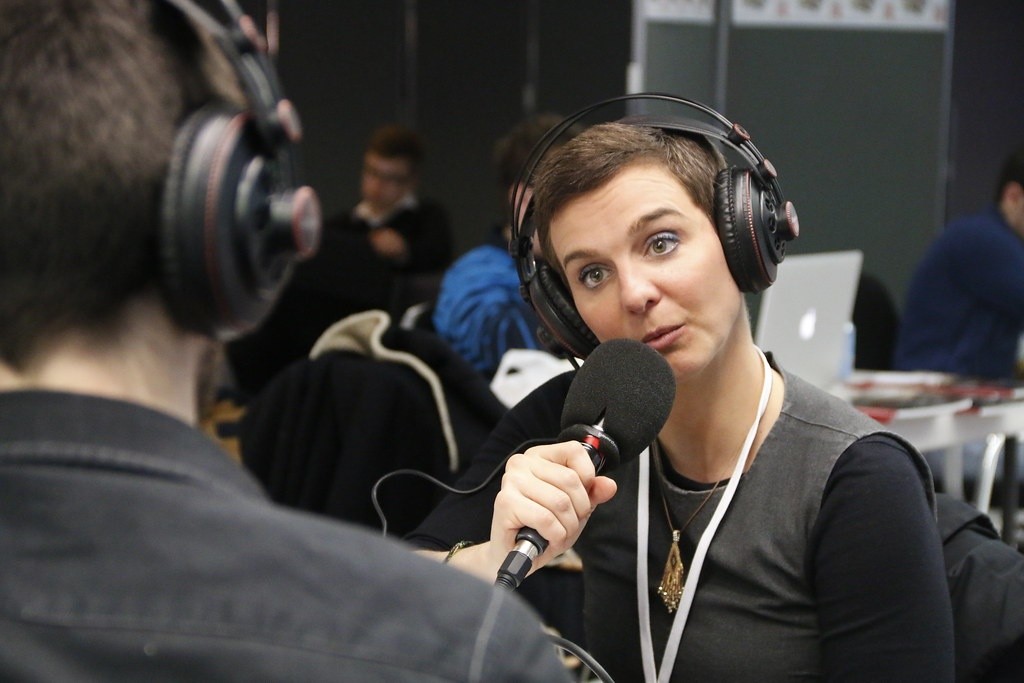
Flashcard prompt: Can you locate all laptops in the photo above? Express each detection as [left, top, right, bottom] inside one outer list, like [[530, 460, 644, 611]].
[[754, 248, 865, 391]]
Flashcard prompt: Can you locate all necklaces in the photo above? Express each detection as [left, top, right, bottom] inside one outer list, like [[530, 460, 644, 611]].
[[648, 436, 749, 615]]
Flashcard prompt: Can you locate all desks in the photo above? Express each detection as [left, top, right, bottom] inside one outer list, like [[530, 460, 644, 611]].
[[887, 406, 1024, 551]]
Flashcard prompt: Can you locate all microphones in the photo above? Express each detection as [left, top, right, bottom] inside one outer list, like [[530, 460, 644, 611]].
[[486, 338, 677, 585]]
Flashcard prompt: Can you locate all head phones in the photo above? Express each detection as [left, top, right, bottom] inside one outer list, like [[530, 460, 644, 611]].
[[129, 0, 320, 343], [509, 90, 800, 358]]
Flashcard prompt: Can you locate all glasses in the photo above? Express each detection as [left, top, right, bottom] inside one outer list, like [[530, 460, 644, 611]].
[[361, 162, 413, 185]]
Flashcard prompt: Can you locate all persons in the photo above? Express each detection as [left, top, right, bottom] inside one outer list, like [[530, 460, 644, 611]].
[[894, 152, 1024, 484], [0, 0, 572, 683], [412, 118, 956, 683], [222, 115, 573, 425]]
